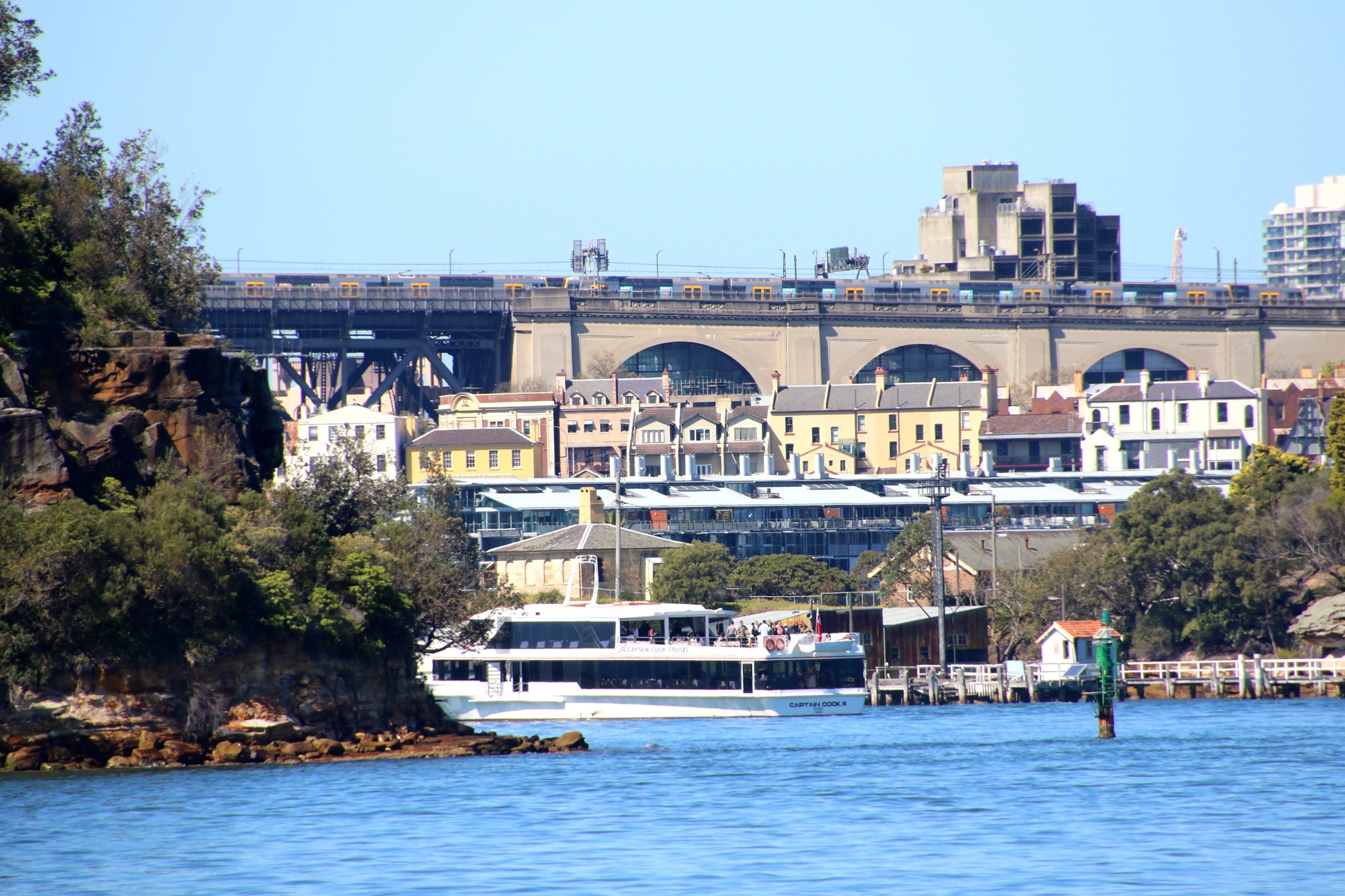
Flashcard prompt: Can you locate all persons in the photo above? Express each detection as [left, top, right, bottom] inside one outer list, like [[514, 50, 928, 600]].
[[626, 625, 639, 640], [716, 617, 726, 641], [727, 621, 737, 641], [737, 620, 748, 637], [747, 619, 813, 648], [648, 626, 656, 644]]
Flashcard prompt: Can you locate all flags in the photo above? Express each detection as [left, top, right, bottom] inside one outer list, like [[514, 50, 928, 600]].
[[817, 609, 822, 642]]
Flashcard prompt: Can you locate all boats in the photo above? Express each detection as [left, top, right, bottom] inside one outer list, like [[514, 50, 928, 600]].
[[413, 601, 871, 725]]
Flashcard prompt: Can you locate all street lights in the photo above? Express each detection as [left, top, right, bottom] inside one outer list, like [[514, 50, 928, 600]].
[[236, 247, 244, 273], [812, 250, 819, 280], [656, 248, 664, 278], [1109, 250, 1119, 282], [883, 251, 890, 280], [778, 249, 787, 278], [1033, 248, 1041, 279], [1211, 245, 1222, 285], [449, 247, 456, 278]]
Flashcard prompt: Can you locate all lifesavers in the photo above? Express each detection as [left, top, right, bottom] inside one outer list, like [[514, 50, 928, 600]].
[[844, 635, 852, 640], [775, 638, 784, 650], [765, 639, 775, 651]]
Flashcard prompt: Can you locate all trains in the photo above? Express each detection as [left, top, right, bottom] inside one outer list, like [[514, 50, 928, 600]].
[[170, 270, 1312, 316]]
